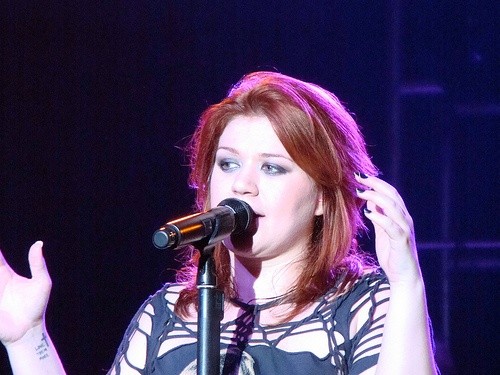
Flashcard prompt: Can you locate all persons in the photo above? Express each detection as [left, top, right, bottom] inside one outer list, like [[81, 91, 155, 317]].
[[0, 72, 441, 375]]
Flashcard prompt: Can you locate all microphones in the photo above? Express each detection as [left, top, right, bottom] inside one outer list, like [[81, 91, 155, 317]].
[[152, 198, 254, 250]]
